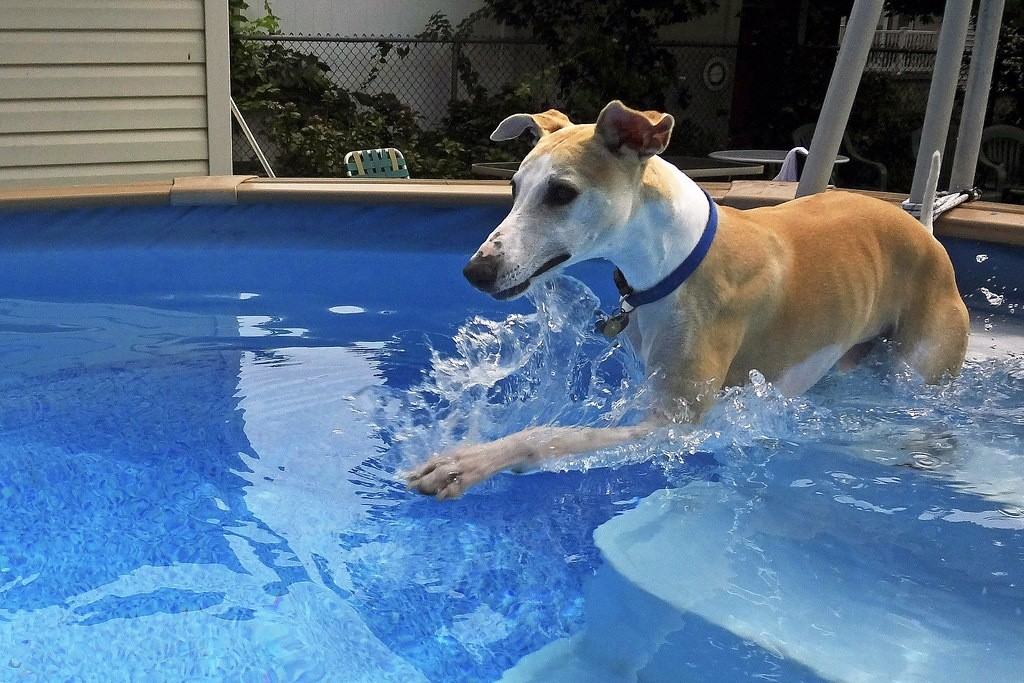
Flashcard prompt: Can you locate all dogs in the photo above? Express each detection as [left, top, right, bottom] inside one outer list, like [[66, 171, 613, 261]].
[[400, 96, 972, 502]]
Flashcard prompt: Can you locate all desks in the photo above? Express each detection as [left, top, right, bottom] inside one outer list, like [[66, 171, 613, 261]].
[[709, 149, 849, 181], [471, 154, 769, 181]]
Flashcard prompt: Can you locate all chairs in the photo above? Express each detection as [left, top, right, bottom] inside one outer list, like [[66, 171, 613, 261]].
[[794, 122, 888, 191], [344, 148, 411, 179], [978, 124, 1024, 204], [912, 122, 1004, 202]]
